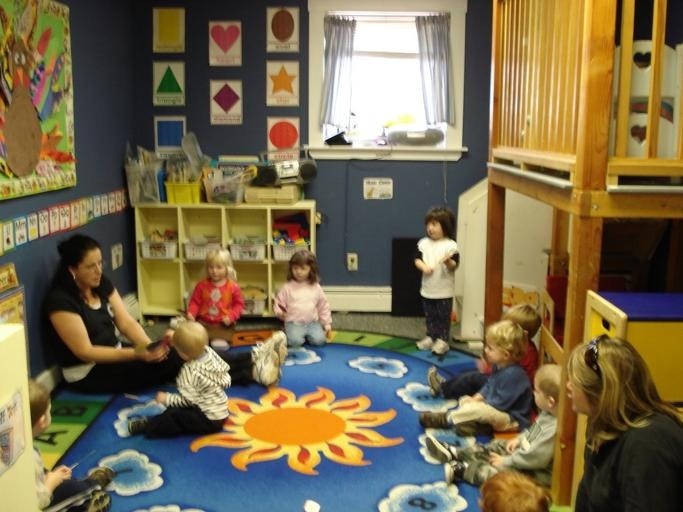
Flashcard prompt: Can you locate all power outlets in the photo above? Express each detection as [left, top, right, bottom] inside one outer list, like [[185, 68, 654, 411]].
[[347, 252, 358, 271]]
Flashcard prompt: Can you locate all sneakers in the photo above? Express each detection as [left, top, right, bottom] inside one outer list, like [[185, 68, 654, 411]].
[[419, 410, 451, 429], [431, 365, 447, 384], [251, 330, 287, 363], [428, 368, 440, 397], [415, 335, 433, 351], [426, 434, 456, 463], [444, 460, 466, 485], [255, 336, 288, 388], [87, 465, 114, 488], [455, 420, 493, 437], [82, 490, 111, 512], [128, 416, 146, 434], [431, 338, 450, 355]]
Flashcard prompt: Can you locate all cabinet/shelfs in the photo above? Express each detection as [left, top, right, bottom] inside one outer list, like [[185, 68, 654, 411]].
[[135, 199, 317, 317]]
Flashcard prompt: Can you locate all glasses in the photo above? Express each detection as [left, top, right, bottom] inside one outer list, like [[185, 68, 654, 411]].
[[87, 260, 103, 272], [584, 333, 609, 380]]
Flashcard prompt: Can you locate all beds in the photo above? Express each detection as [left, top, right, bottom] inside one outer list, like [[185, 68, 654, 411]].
[[484, 0, 682, 511]]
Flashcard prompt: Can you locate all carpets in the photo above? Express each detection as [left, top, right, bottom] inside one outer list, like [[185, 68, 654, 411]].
[[32, 328, 542, 512]]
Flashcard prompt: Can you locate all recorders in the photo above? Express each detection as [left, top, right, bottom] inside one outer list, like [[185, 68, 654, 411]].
[[250, 147, 318, 188]]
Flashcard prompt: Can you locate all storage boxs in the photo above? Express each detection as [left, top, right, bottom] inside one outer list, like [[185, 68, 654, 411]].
[[230, 240, 264, 261], [183, 240, 219, 261], [273, 243, 311, 263], [242, 293, 264, 316], [203, 179, 244, 205], [165, 182, 200, 203], [112, 244, 124, 270], [138, 240, 177, 259], [571, 288, 682, 512]]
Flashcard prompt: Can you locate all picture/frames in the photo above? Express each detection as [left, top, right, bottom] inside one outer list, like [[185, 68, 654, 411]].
[[209, 22, 243, 67], [267, 6, 299, 54], [152, 7, 185, 52], [268, 116, 300, 160], [153, 60, 186, 106], [267, 60, 299, 108], [210, 79, 243, 125], [155, 116, 187, 151]]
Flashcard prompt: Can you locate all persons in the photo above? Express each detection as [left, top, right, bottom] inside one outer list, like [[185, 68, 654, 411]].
[[127, 319, 232, 441], [426, 303, 542, 401], [28, 378, 116, 512], [417, 319, 535, 438], [185, 247, 245, 350], [46, 232, 288, 393], [565, 334, 683, 512], [273, 248, 333, 348], [424, 362, 563, 488], [478, 467, 554, 512], [412, 205, 461, 355]]
